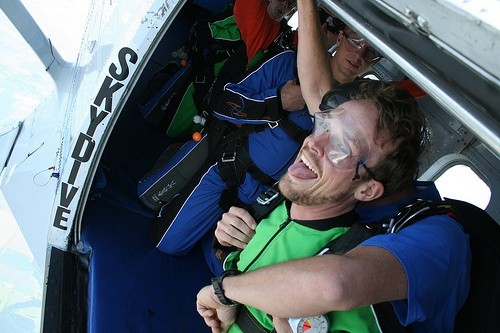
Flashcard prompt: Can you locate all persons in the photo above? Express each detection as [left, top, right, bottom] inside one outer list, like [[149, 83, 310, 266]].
[[194, 75, 433, 332], [139, 0, 486, 330]]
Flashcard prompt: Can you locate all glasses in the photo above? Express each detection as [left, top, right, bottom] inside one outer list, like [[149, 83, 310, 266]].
[[316, 7, 347, 35], [340, 28, 382, 64], [277, 0, 298, 14], [313, 106, 379, 183]]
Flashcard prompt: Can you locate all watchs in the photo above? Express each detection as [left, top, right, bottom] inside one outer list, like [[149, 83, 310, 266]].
[[207, 268, 246, 308]]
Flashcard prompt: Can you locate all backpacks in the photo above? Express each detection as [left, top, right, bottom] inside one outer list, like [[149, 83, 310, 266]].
[[380, 198, 500, 333]]
